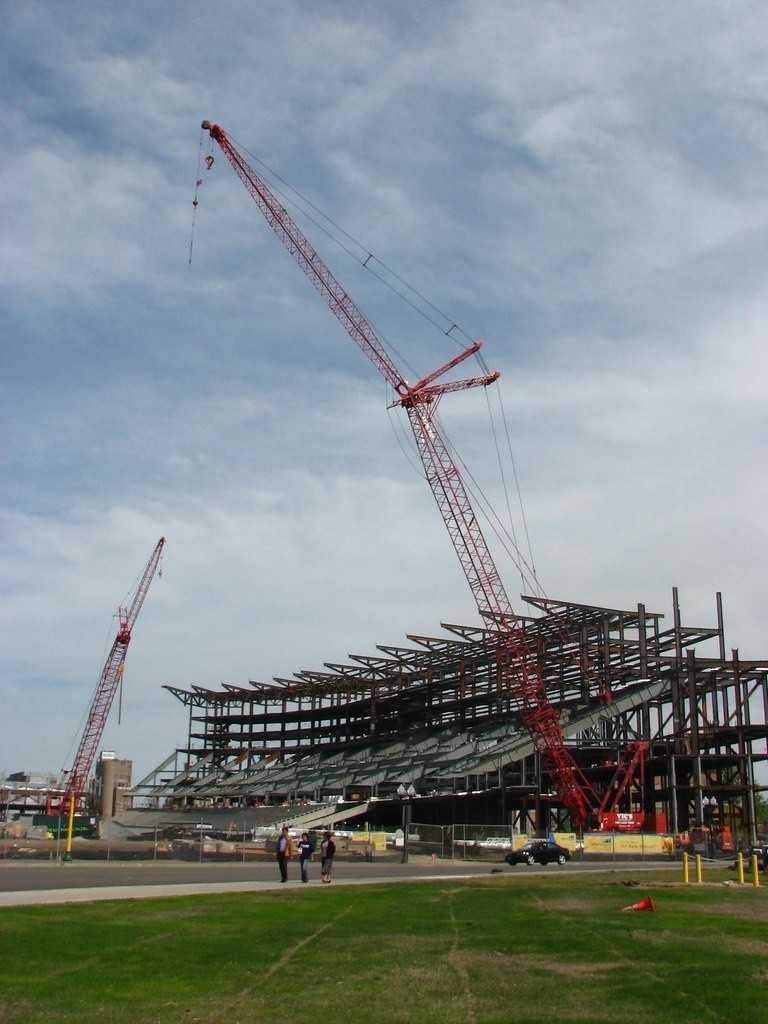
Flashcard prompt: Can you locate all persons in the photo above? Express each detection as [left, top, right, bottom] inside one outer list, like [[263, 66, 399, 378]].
[[273, 826, 293, 884], [320, 831, 336, 883], [292, 832, 314, 883]]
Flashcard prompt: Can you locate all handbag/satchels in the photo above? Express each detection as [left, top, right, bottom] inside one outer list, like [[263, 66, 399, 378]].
[[297, 846, 303, 854]]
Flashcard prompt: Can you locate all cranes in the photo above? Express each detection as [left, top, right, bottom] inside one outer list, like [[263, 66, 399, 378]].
[[196, 116, 668, 835], [32, 533, 167, 839]]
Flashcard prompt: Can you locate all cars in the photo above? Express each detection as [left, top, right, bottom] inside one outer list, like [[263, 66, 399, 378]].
[[505, 841, 573, 866]]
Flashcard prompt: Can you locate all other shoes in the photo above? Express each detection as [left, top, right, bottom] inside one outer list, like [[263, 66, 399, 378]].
[[280, 880, 286, 883], [302, 878, 309, 883], [322, 879, 332, 883]]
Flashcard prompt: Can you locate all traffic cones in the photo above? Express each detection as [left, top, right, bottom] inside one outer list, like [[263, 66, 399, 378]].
[[623, 895, 658, 913]]
